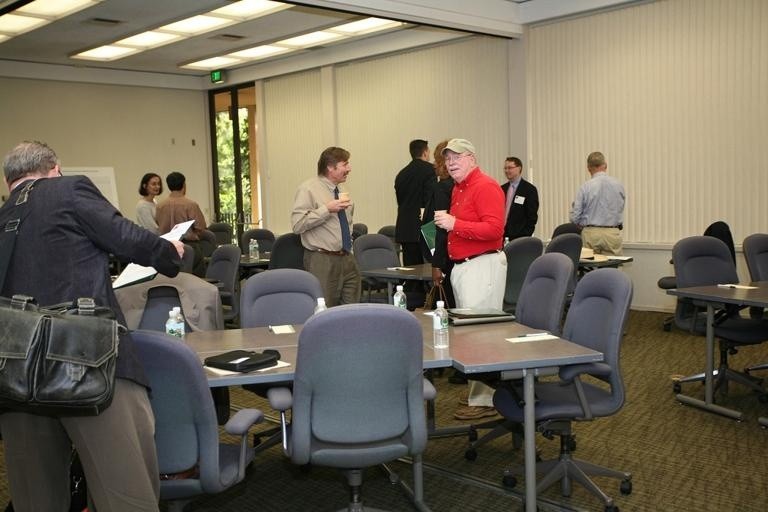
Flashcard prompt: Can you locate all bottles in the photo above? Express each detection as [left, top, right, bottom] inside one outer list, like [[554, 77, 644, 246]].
[[313, 297, 327, 315], [503, 236, 510, 247], [434, 346, 450, 360], [432, 299, 448, 347], [249, 238, 259, 259], [393, 284, 408, 310], [166, 305, 186, 343]]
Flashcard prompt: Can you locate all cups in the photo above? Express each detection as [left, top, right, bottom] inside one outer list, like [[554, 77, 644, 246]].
[[337, 193, 350, 201], [434, 209, 447, 221]]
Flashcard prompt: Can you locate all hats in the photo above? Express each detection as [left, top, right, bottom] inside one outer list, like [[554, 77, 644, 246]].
[[440, 136, 474, 153]]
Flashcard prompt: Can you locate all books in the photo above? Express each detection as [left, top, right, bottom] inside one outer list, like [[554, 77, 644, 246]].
[[447, 309, 515, 328]]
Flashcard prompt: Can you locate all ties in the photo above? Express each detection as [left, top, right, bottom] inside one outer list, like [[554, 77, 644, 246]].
[[504, 184, 513, 229], [333, 185, 354, 256]]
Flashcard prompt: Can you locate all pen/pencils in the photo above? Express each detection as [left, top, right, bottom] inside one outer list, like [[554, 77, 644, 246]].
[[385, 268, 399, 270], [716, 284, 735, 288], [517, 333, 548, 337], [268, 325, 272, 331]]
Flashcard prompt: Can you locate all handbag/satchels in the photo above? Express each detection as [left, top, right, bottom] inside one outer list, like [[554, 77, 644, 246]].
[[0, 296, 119, 416]]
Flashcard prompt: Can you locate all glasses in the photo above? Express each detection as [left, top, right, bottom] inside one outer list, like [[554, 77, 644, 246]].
[[444, 154, 471, 160], [501, 164, 519, 172]]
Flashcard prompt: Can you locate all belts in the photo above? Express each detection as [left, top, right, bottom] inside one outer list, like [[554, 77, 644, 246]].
[[586, 221, 620, 231], [303, 246, 347, 257], [448, 247, 503, 266]]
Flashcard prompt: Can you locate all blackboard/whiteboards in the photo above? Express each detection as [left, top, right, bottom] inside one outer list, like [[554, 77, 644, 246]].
[[58, 166, 120, 211]]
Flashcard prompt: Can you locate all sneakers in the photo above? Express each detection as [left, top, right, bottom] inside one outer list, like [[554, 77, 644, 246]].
[[446, 372, 505, 424]]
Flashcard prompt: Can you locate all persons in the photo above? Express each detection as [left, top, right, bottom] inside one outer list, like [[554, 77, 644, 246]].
[[571, 152, 626, 271], [135, 173, 163, 236], [499, 157, 539, 248], [434, 138, 508, 420], [419, 140, 456, 309], [156, 172, 207, 278], [394, 140, 438, 311], [0, 141, 185, 512], [290, 147, 361, 307]]
